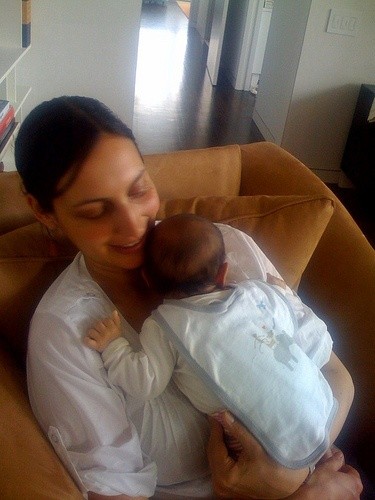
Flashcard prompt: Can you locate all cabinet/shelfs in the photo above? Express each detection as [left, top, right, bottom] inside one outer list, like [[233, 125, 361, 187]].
[[0, 46, 33, 159]]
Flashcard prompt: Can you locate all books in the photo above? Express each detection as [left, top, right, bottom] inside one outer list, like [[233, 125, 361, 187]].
[[0, 97, 17, 152]]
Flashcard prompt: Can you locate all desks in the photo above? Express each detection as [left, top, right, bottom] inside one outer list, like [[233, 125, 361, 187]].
[[339, 83, 375, 198]]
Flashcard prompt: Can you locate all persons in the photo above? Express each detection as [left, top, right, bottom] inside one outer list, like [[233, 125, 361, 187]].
[[80, 215, 338, 469], [14, 96, 363, 499]]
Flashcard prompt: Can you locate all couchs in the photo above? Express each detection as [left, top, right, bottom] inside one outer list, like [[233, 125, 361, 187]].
[[0, 141, 375, 500]]
